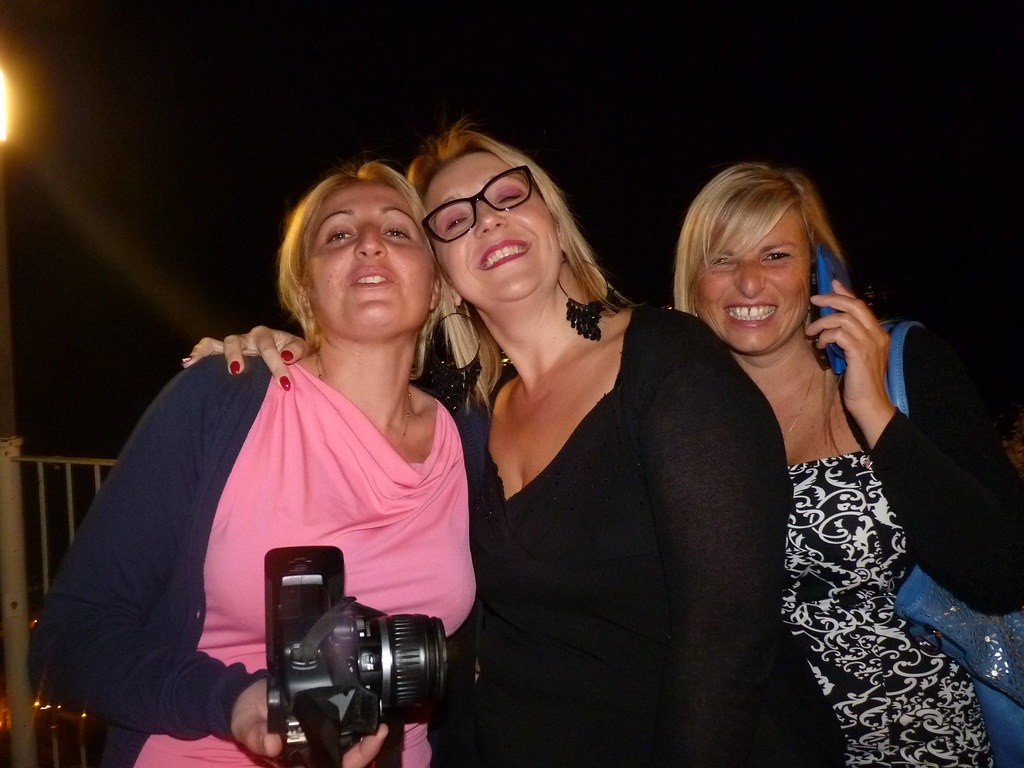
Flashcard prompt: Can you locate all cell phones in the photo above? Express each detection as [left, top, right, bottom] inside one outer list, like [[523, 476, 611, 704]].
[[810, 244, 848, 375]]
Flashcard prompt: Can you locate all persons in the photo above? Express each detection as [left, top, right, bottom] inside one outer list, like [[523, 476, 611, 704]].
[[178, 126, 851, 767], [28, 161, 482, 768], [665, 159, 1022, 768]]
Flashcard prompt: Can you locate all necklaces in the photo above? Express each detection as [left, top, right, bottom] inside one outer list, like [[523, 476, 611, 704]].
[[305, 355, 417, 456]]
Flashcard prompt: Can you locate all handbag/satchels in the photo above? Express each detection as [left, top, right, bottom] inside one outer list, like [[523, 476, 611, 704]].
[[866, 318, 1024, 766]]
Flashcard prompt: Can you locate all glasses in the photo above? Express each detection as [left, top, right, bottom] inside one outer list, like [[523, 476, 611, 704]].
[[422, 164, 543, 243]]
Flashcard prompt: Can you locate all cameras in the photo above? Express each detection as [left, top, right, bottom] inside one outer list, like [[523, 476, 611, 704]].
[[266, 546, 450, 754]]
